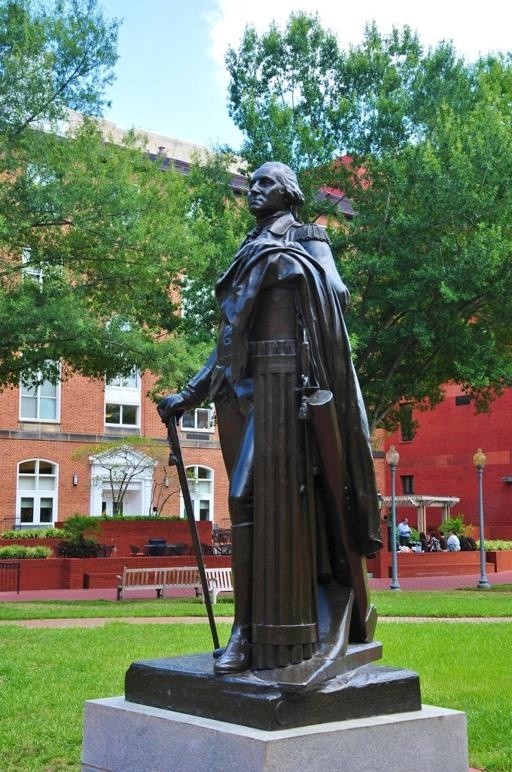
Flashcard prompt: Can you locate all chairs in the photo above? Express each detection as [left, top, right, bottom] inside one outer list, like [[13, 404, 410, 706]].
[[131, 537, 212, 556]]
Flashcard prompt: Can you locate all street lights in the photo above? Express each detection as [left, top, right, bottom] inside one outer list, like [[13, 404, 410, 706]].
[[472, 448, 492, 591], [383, 444, 401, 591]]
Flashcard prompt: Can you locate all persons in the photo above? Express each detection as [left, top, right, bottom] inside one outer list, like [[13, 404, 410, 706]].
[[153, 159, 385, 678], [397, 517, 412, 546], [416, 530, 462, 552]]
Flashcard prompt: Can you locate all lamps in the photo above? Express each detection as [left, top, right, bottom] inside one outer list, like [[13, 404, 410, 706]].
[[165, 477, 169, 487], [73, 475, 78, 486]]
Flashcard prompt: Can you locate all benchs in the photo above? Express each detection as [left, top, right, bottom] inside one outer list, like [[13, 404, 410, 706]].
[[116, 567, 203, 601], [204, 568, 233, 604]]
